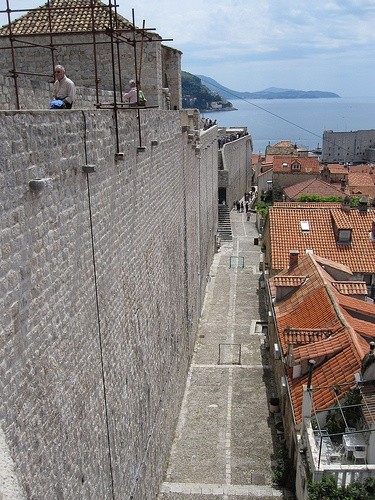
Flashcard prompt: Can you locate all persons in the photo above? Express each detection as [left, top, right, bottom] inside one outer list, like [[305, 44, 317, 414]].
[[173, 105, 179, 110], [202, 118, 218, 130], [123, 79, 147, 106], [218, 130, 249, 148], [49, 65, 76, 109], [231, 189, 255, 213]]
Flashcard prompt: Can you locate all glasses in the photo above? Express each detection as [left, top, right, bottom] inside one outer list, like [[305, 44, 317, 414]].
[[54, 71, 60, 73]]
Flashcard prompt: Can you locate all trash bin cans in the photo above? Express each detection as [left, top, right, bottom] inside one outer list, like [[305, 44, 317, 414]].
[[253, 238, 258, 245]]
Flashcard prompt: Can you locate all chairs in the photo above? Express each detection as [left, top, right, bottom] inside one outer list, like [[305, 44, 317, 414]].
[[344, 428, 356, 434], [327, 451, 342, 464], [352, 445, 368, 465]]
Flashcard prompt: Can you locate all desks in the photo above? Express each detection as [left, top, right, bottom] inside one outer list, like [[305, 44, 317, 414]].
[[342, 433, 367, 462]]
